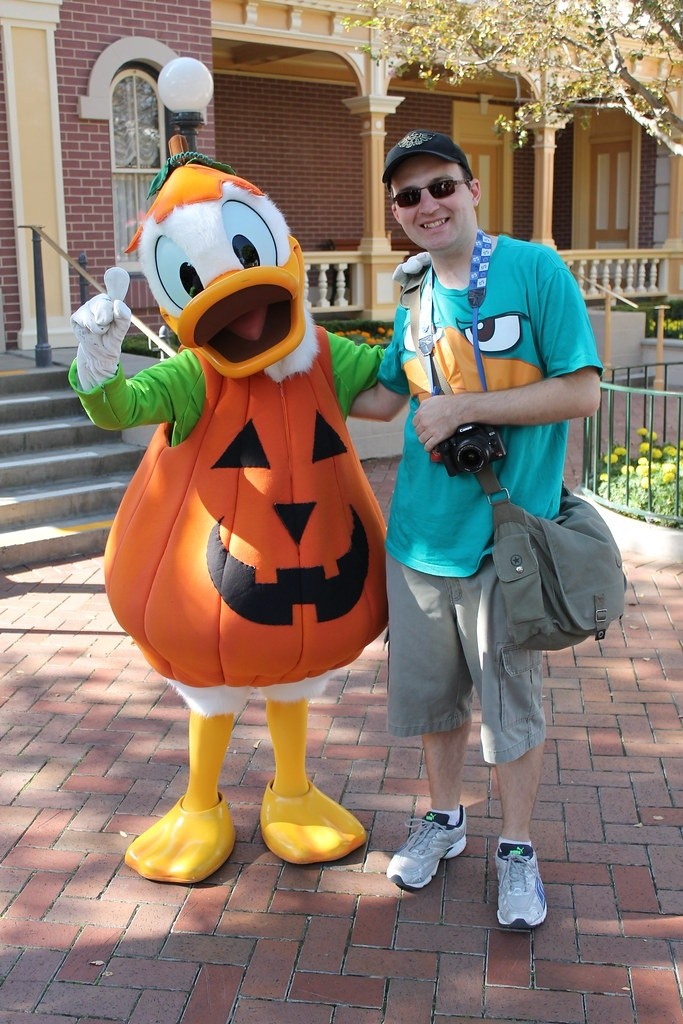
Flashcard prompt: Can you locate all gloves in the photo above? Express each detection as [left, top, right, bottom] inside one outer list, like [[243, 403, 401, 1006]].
[[69, 267, 131, 394]]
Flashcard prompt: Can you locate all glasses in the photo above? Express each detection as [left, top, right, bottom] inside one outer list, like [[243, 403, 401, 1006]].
[[392, 177, 470, 208]]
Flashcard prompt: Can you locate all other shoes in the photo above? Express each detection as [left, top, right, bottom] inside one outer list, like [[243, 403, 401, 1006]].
[[125, 791, 236, 885], [259, 775, 366, 863]]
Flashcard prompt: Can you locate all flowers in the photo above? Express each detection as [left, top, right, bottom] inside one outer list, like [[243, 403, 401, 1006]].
[[596, 426, 682, 518], [649, 318, 683, 340]]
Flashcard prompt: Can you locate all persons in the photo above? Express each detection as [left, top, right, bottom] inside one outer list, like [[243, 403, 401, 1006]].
[[349, 128, 608, 931]]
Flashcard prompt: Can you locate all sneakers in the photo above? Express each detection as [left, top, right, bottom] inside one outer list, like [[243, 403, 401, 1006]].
[[494, 841, 547, 928], [386, 804, 468, 890]]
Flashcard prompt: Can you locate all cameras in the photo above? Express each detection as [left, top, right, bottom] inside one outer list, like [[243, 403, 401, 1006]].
[[438, 423, 506, 477]]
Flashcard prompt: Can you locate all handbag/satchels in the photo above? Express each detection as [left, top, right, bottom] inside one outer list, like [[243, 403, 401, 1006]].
[[488, 484, 631, 647]]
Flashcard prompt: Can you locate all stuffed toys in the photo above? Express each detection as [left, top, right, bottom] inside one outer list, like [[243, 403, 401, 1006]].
[[67, 130, 432, 883]]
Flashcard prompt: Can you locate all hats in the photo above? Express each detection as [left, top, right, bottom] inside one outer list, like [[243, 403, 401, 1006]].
[[380, 128, 473, 193], [124, 135, 267, 257]]
